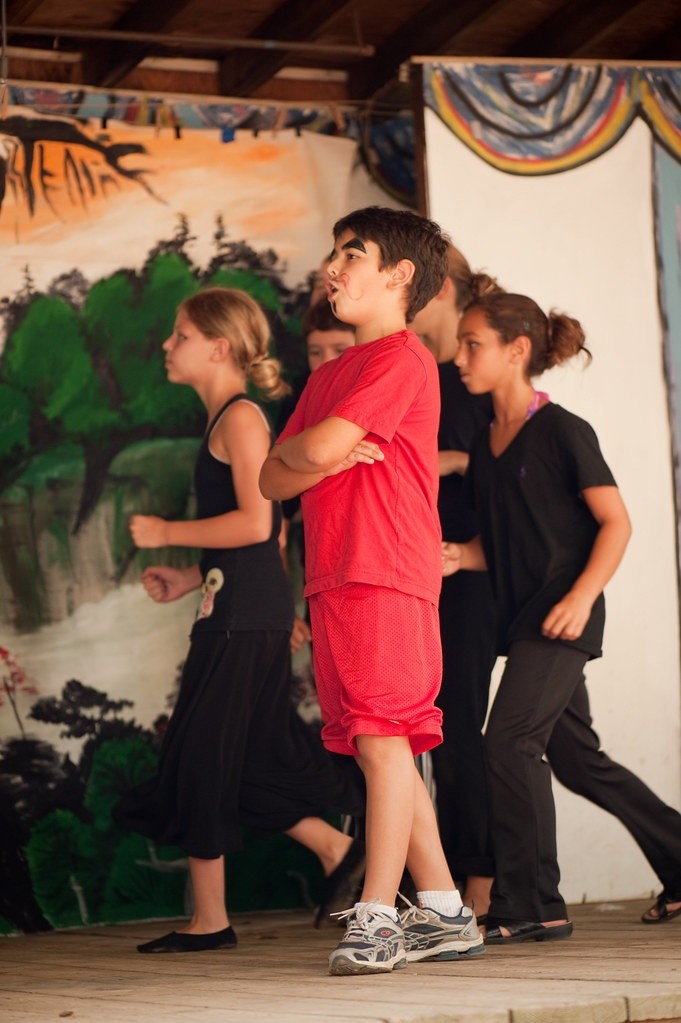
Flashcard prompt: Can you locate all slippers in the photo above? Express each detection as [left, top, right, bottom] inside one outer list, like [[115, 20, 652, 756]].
[[642, 897, 681, 921], [477, 910, 573, 944]]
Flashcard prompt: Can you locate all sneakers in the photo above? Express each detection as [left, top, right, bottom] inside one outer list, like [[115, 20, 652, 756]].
[[395, 890, 485, 962], [328, 898, 407, 974]]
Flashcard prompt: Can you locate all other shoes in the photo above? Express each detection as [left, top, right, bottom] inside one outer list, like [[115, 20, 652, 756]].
[[137, 924, 237, 953], [315, 837, 367, 930]]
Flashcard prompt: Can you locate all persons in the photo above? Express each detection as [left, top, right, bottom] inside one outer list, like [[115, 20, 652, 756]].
[[278, 233, 681, 946], [259, 204, 486, 977], [129, 285, 367, 954]]
